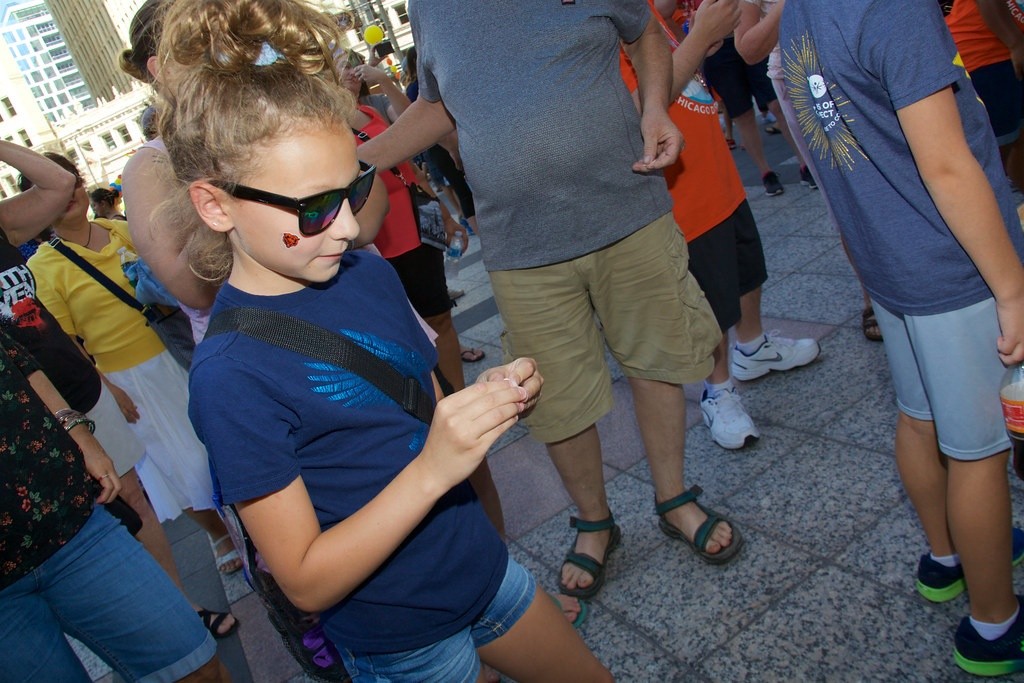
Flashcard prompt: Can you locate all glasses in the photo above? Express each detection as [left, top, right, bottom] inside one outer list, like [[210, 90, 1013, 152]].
[[208, 160, 377, 238]]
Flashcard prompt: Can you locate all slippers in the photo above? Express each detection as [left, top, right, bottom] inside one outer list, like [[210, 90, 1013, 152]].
[[460, 347, 486, 362]]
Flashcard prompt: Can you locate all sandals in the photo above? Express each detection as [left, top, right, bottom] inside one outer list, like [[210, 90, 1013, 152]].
[[213, 533, 244, 576], [197, 607, 240, 639], [557, 508, 621, 598], [863, 306, 883, 342], [544, 592, 589, 629], [764, 126, 782, 134], [653, 484, 745, 565]]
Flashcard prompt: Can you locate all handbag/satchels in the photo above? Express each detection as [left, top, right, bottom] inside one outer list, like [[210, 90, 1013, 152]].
[[409, 181, 446, 251], [148, 308, 196, 372], [244, 540, 353, 683]]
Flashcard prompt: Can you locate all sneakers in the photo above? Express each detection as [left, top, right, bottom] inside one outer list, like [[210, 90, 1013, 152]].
[[952, 594, 1024, 676], [761, 170, 785, 197], [726, 139, 737, 149], [700, 384, 761, 450], [800, 163, 818, 189], [460, 218, 476, 236], [916, 527, 1024, 603], [731, 329, 821, 382]]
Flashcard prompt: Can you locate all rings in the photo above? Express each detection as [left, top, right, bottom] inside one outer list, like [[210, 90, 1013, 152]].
[[98, 474, 109, 482]]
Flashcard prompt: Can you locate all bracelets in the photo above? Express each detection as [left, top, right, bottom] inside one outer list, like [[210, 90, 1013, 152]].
[[56, 408, 97, 435]]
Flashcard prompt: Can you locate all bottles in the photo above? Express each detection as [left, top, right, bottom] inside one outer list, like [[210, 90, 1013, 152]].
[[998, 363, 1024, 482], [443, 231, 463, 280], [115, 246, 140, 286]]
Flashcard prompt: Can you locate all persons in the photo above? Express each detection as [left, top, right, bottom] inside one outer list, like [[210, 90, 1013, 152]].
[[616, 0, 1024, 450], [16, 152, 245, 576], [89, 188, 126, 221], [358, 0, 745, 596], [0, 139, 240, 640], [122, 0, 615, 683], [0, 332, 232, 683], [777, 0, 1024, 676]]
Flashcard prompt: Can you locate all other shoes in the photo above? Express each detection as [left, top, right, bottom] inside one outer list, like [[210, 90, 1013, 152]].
[[447, 288, 464, 299]]
